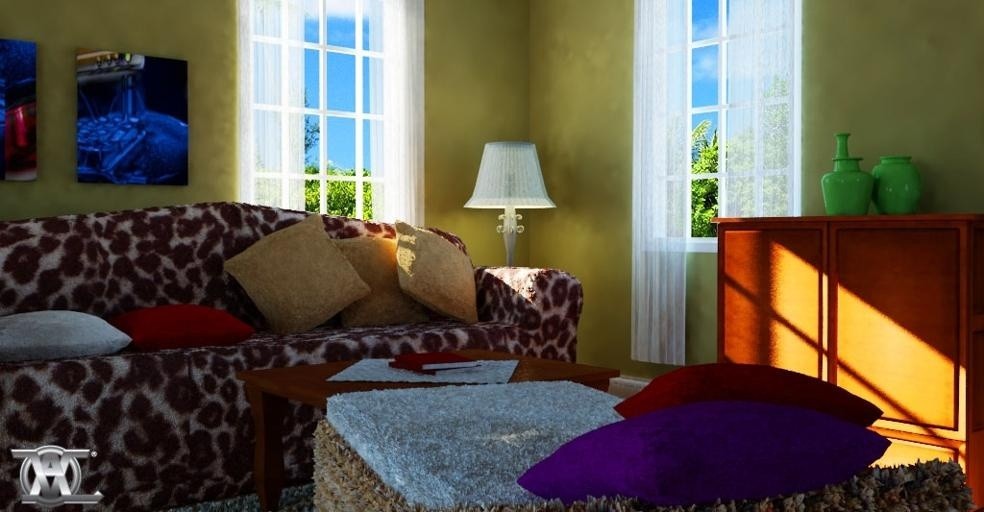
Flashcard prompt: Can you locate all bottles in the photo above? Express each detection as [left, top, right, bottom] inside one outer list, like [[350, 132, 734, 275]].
[[820, 131, 925, 216]]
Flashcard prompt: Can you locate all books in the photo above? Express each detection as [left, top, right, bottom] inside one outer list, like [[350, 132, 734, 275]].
[[394, 348, 482, 371], [388, 362, 483, 378]]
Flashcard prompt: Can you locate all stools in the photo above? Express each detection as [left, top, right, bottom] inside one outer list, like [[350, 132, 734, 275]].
[[312, 380, 628, 511]]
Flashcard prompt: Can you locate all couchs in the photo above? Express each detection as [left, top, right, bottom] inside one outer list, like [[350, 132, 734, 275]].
[[0, 201, 581, 512]]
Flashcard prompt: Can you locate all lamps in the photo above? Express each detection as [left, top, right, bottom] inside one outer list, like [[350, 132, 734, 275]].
[[465, 140, 557, 263]]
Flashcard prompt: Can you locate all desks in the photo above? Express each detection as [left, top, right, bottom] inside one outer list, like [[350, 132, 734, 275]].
[[237, 353, 620, 510]]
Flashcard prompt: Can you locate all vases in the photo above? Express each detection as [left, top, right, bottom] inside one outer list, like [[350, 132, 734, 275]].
[[820, 125, 922, 215]]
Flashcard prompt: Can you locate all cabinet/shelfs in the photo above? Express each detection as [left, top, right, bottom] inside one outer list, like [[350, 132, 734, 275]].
[[709, 214, 982, 506]]
